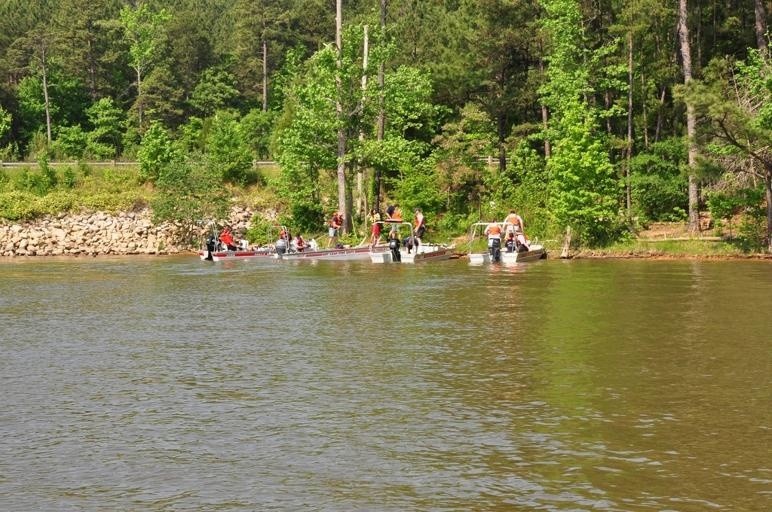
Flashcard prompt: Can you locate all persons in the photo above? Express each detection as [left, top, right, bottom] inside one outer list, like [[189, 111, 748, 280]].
[[279, 227, 292, 242], [327, 211, 344, 250], [293, 232, 311, 254], [503, 210, 524, 240], [367, 207, 382, 246], [385, 204, 403, 240], [412, 207, 426, 241], [221, 226, 241, 251], [504, 232, 521, 253], [205, 230, 218, 260], [483, 218, 503, 263]]
